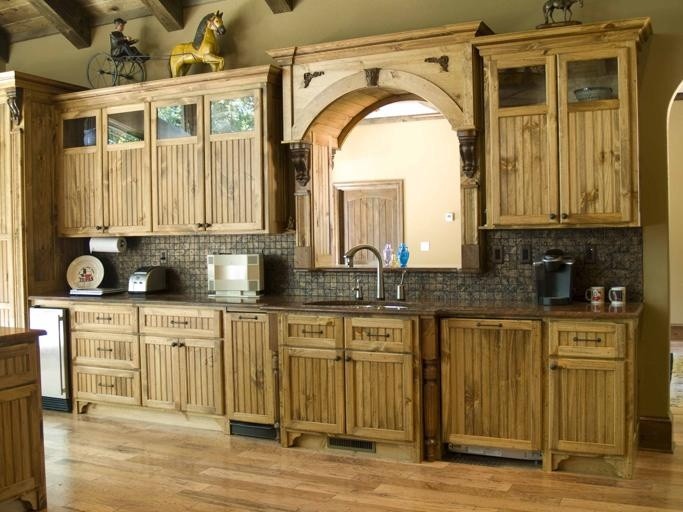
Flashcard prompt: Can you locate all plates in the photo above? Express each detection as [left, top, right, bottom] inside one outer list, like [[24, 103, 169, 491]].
[[65, 255, 105, 289]]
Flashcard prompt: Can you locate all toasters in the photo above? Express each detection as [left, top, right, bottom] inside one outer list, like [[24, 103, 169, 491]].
[[127, 266, 166, 294]]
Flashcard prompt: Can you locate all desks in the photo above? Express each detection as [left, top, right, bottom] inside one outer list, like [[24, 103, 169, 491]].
[[0, 326, 48, 512]]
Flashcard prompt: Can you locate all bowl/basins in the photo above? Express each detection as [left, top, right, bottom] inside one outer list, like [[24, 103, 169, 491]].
[[573, 87, 611, 102]]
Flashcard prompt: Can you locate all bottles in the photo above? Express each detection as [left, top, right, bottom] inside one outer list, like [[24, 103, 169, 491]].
[[382, 241, 410, 267]]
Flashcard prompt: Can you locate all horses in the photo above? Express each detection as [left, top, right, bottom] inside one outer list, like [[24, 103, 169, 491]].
[[169, 10, 226, 77], [543, 0, 584, 25]]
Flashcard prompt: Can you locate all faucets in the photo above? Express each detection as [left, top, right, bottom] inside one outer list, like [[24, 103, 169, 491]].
[[343, 244, 384, 299]]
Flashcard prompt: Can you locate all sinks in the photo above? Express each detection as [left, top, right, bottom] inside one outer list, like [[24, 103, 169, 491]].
[[303, 300, 410, 309]]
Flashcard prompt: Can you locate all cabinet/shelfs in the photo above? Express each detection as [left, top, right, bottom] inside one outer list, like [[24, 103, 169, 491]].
[[470, 16, 653, 230], [543, 315, 640, 480], [223, 311, 277, 425], [137, 306, 225, 434], [151, 65, 286, 237], [440, 317, 542, 463], [1, 69, 97, 331], [67, 301, 141, 414], [51, 82, 151, 237], [276, 310, 422, 465]]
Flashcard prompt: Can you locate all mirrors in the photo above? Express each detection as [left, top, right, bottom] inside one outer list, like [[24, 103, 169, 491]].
[[265, 17, 494, 275]]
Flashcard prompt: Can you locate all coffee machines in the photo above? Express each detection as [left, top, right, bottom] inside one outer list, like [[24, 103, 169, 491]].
[[532, 248, 575, 307]]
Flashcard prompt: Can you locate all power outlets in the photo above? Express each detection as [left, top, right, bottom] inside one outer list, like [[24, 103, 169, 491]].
[[584, 244, 595, 265], [492, 245, 503, 264], [519, 245, 532, 263]]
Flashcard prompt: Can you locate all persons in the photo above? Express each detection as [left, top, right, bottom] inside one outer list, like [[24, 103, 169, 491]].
[[110, 18, 150, 63]]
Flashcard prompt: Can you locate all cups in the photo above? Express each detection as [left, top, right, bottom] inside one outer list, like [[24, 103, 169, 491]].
[[83, 128, 95, 145], [607, 288, 625, 307], [584, 286, 606, 306]]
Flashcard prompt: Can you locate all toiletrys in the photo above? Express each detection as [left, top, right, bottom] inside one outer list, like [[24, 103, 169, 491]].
[[398, 244, 410, 267], [383, 243, 393, 267]]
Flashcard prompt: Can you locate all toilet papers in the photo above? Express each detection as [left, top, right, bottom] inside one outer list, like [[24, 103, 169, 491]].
[[88, 237, 126, 253]]
